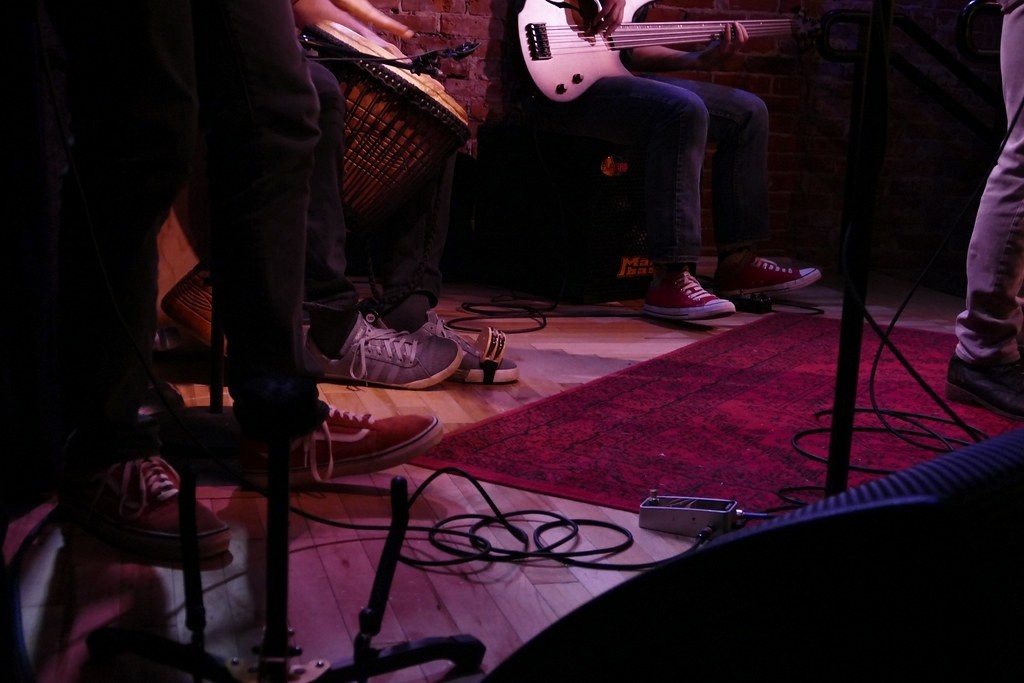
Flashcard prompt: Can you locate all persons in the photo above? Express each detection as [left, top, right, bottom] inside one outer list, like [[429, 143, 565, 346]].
[[287, 0, 519, 391], [944, 0, 1024, 421], [0, 0, 441, 560], [526, 0, 821, 320]]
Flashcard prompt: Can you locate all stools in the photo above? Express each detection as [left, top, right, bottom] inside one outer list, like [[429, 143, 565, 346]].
[[472, 124, 656, 307]]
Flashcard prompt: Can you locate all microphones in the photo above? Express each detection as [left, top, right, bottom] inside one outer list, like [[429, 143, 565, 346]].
[[453, 40, 481, 60]]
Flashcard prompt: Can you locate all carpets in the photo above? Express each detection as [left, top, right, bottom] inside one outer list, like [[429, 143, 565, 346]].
[[407, 310, 1024, 536]]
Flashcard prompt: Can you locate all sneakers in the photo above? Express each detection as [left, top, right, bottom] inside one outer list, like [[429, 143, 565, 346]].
[[367, 299, 519, 385], [643, 265, 735, 320], [240, 398, 441, 489], [303, 307, 463, 389], [67, 453, 230, 558], [714, 249, 822, 297]]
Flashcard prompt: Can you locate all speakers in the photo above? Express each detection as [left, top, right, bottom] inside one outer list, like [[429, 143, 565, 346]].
[[477, 425, 1024, 683]]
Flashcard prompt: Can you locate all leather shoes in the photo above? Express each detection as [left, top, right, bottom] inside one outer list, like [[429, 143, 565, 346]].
[[943, 350, 1024, 422]]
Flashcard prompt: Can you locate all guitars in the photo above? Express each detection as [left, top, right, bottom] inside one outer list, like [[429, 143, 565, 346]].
[[507, 0, 822, 112]]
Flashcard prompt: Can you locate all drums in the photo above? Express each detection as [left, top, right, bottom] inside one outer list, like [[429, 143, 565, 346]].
[[158, 19, 473, 361]]
